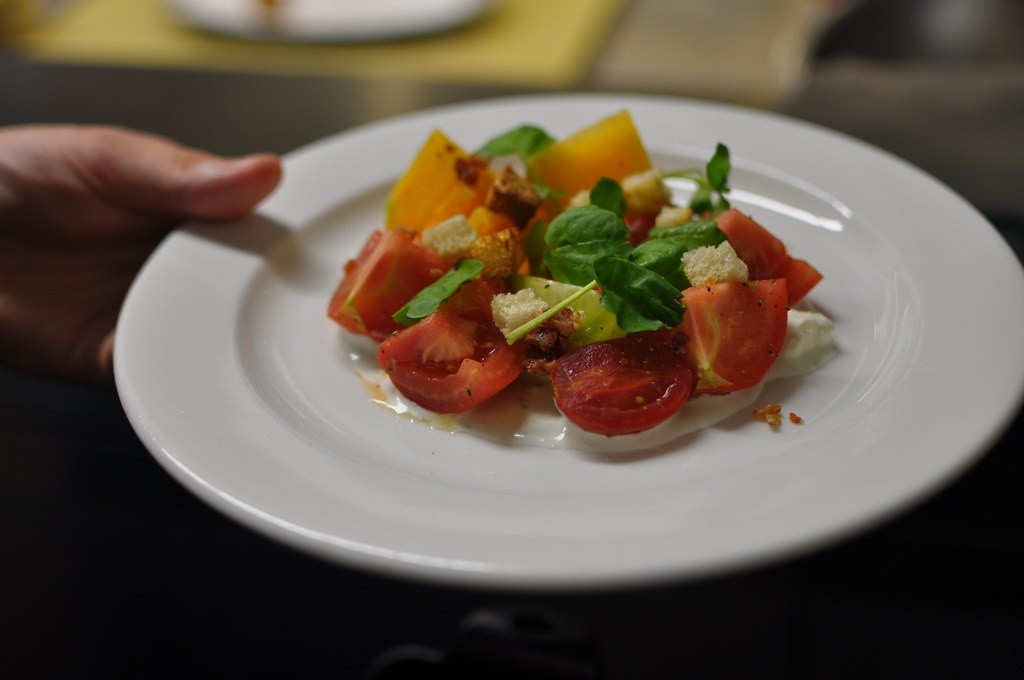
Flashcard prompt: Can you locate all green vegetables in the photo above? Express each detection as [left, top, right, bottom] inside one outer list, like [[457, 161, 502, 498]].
[[391, 125, 732, 344]]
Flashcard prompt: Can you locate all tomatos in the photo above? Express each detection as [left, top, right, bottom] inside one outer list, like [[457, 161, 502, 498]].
[[325, 206, 823, 437]]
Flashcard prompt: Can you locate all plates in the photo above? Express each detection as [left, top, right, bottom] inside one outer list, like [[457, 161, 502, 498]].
[[180, 0, 485, 42], [114, 96, 1023, 586]]
